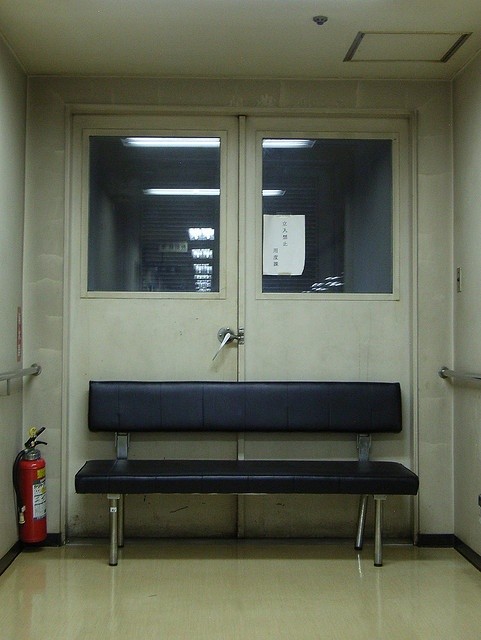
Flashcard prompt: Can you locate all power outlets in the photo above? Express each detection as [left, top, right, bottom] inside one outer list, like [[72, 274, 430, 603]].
[[457, 267, 462, 293]]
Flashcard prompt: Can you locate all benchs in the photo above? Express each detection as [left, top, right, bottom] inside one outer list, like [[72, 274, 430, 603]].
[[75, 381, 418, 567]]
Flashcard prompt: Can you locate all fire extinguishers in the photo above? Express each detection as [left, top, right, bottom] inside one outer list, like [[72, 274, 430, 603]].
[[13, 427, 48, 543]]
